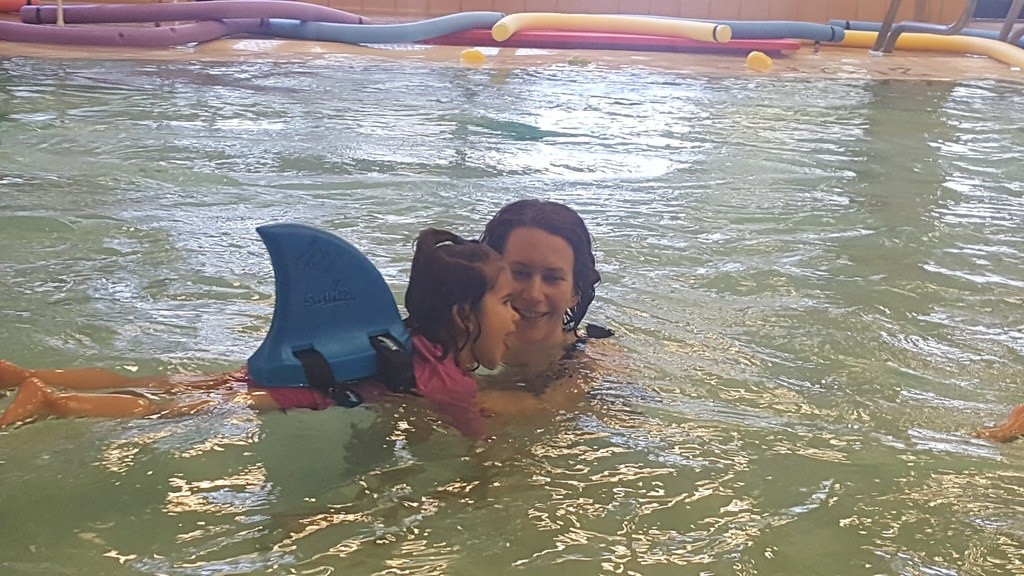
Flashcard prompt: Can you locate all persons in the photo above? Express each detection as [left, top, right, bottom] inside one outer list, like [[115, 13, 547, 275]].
[[0, 226, 519, 446], [473, 200, 625, 382]]
